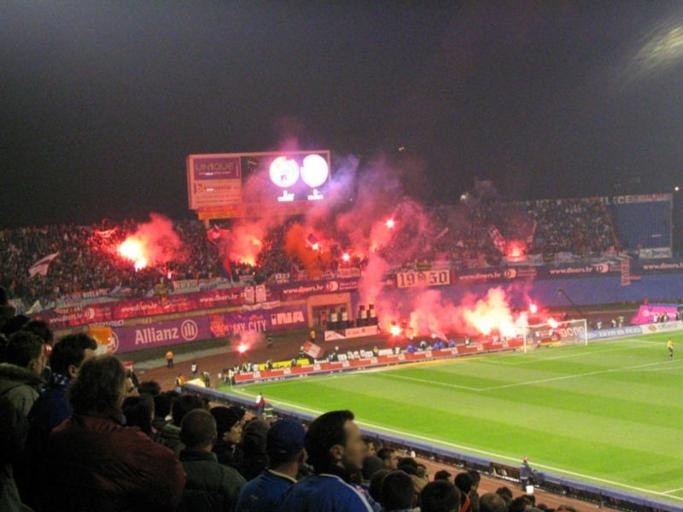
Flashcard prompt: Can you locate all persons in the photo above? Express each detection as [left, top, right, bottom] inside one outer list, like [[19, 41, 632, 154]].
[[0, 407, 577, 512], [0, 216, 274, 408], [666, 336, 675, 360], [652, 310, 682, 324], [275, 192, 625, 368]]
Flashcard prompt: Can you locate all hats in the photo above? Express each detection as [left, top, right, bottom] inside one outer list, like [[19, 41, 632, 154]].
[[210, 407, 239, 434], [266, 418, 305, 454]]
